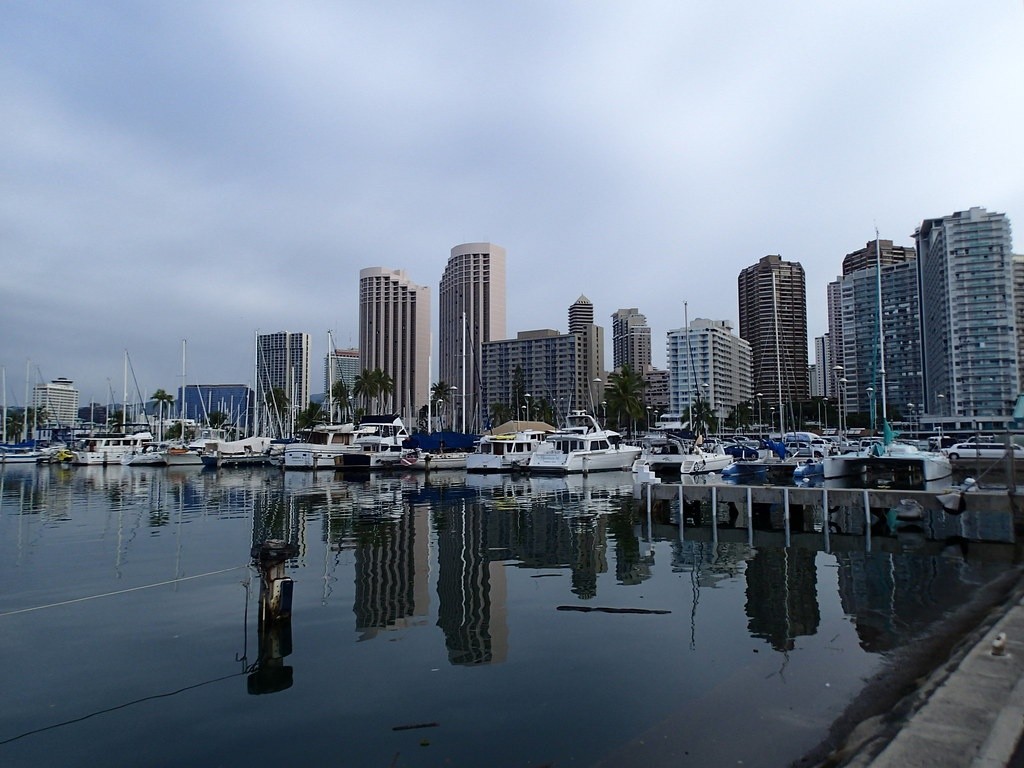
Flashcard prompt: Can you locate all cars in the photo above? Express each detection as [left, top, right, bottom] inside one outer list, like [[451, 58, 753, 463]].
[[685, 431, 886, 463], [938, 442, 1024, 459]]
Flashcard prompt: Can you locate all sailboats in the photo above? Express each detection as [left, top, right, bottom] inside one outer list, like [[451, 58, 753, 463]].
[[0, 337, 404, 469]]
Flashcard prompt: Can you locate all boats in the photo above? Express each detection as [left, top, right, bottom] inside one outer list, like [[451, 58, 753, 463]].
[[719, 460, 797, 482], [465, 433, 546, 473], [526, 410, 643, 474], [631, 439, 734, 483], [403, 448, 470, 469]]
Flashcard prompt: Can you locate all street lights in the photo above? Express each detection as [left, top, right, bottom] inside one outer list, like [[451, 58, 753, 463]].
[[702, 383, 709, 399], [716, 400, 723, 439], [593, 377, 609, 427], [521, 393, 532, 424], [938, 393, 947, 438], [770, 406, 775, 433], [822, 397, 828, 432], [757, 392, 764, 441], [646, 406, 659, 432], [434, 385, 457, 435], [907, 402, 913, 438], [833, 364, 847, 446], [867, 387, 875, 437]]
